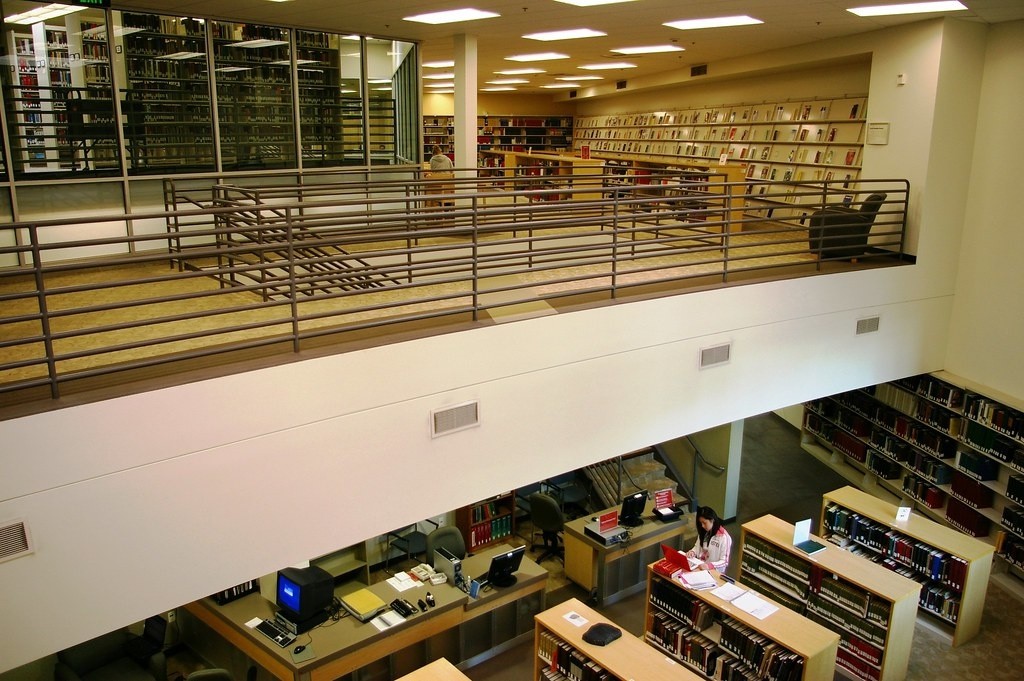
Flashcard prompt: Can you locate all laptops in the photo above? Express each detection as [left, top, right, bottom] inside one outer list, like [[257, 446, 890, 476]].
[[661, 544, 704, 571]]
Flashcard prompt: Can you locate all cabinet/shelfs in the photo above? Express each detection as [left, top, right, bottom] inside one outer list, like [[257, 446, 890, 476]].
[[308, 539, 371, 598], [0, 0, 869, 233], [533, 371, 1024, 681], [456, 489, 514, 553]]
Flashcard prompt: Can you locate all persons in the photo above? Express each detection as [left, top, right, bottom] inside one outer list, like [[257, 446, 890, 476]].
[[686, 506, 732, 572], [431, 145, 454, 173]]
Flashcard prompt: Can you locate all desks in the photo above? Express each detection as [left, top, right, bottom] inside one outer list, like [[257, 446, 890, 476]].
[[425, 179, 487, 223]]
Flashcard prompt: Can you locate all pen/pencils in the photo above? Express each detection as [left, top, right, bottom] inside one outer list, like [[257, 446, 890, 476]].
[[379, 617, 391, 627]]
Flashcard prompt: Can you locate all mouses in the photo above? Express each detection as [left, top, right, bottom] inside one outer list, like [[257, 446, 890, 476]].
[[592, 517, 599, 521], [294, 645, 306, 654]]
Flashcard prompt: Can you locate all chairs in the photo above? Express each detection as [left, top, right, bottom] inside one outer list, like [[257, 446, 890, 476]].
[[425, 525, 465, 569], [514, 481, 563, 545], [545, 470, 591, 513], [433, 183, 456, 225], [529, 494, 565, 565], [426, 173, 450, 180], [384, 519, 440, 577], [187, 668, 233, 681]]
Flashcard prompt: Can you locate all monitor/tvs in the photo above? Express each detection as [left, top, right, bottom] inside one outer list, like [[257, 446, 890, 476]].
[[277, 567, 335, 622], [618, 489, 648, 527], [487, 545, 526, 588]]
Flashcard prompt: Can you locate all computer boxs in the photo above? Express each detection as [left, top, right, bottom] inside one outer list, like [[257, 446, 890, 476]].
[[433, 547, 462, 586], [584, 523, 628, 546]]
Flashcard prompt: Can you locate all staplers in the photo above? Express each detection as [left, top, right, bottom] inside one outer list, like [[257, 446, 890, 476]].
[[417, 599, 428, 612]]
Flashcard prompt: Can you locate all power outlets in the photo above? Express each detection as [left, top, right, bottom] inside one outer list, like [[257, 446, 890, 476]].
[[439, 517, 444, 526], [168, 611, 176, 623]]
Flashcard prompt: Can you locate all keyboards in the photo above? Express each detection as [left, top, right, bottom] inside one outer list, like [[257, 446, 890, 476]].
[[254, 619, 298, 648], [472, 572, 489, 587]]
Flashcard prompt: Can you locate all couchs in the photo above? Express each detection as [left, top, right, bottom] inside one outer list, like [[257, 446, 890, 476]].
[[809, 193, 887, 264], [53, 627, 167, 681]]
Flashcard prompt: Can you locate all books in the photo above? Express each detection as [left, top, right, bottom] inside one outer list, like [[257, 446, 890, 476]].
[[823, 502, 967, 623], [423, 116, 572, 200], [575, 104, 858, 226], [13, 14, 335, 146], [801, 373, 1024, 570], [740, 536, 891, 681], [472, 502, 511, 547], [537, 631, 613, 681], [654, 560, 682, 578], [644, 577, 804, 681]]
